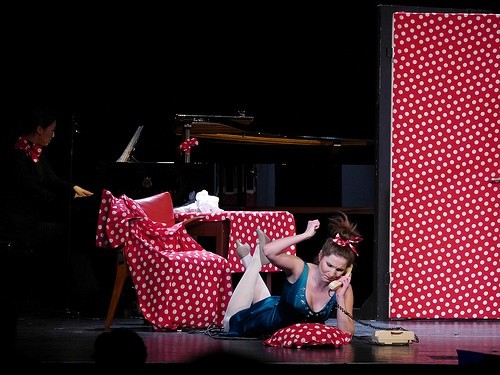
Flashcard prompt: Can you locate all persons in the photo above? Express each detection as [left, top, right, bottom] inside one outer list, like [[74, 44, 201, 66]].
[[221, 209, 362, 338], [8, 108, 99, 291]]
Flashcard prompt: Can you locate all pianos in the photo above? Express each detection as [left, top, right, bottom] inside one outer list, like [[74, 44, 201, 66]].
[[93, 125, 218, 319]]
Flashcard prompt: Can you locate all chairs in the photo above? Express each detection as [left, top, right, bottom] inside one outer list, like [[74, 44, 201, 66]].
[[96, 189, 234, 332]]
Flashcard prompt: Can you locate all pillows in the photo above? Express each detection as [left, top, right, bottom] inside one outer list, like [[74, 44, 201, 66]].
[[264, 323, 353, 348]]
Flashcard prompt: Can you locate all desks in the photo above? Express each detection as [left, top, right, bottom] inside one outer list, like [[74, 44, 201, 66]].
[[174, 210, 296, 296]]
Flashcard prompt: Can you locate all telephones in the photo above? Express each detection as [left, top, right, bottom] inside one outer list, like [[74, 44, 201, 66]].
[[325, 262, 353, 291]]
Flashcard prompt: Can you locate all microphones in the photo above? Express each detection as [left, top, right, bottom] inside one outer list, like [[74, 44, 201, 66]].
[[73, 114, 80, 134]]
